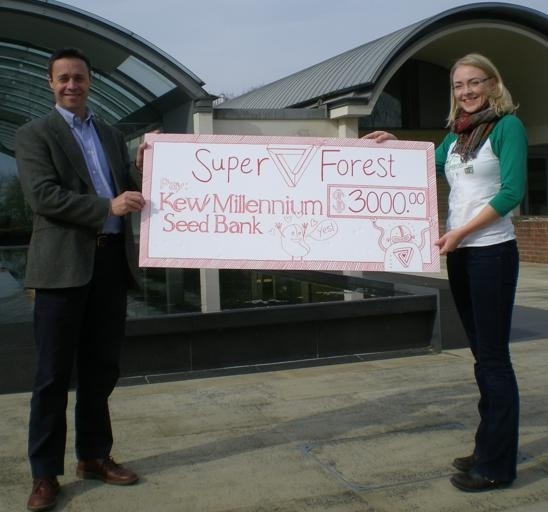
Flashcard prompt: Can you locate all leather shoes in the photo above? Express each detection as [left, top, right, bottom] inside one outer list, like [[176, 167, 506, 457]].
[[27, 476, 61, 510], [452, 452, 480, 472], [76, 456, 138, 485], [450, 468, 512, 492]]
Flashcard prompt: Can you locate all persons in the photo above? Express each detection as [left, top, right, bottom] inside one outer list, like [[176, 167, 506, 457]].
[[16, 48, 146, 510], [359, 53, 529, 492]]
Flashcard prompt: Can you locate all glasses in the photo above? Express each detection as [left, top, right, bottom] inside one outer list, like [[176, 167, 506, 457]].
[[452, 77, 492, 91]]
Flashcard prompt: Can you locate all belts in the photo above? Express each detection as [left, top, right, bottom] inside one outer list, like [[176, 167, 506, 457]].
[[97, 234, 120, 250]]
